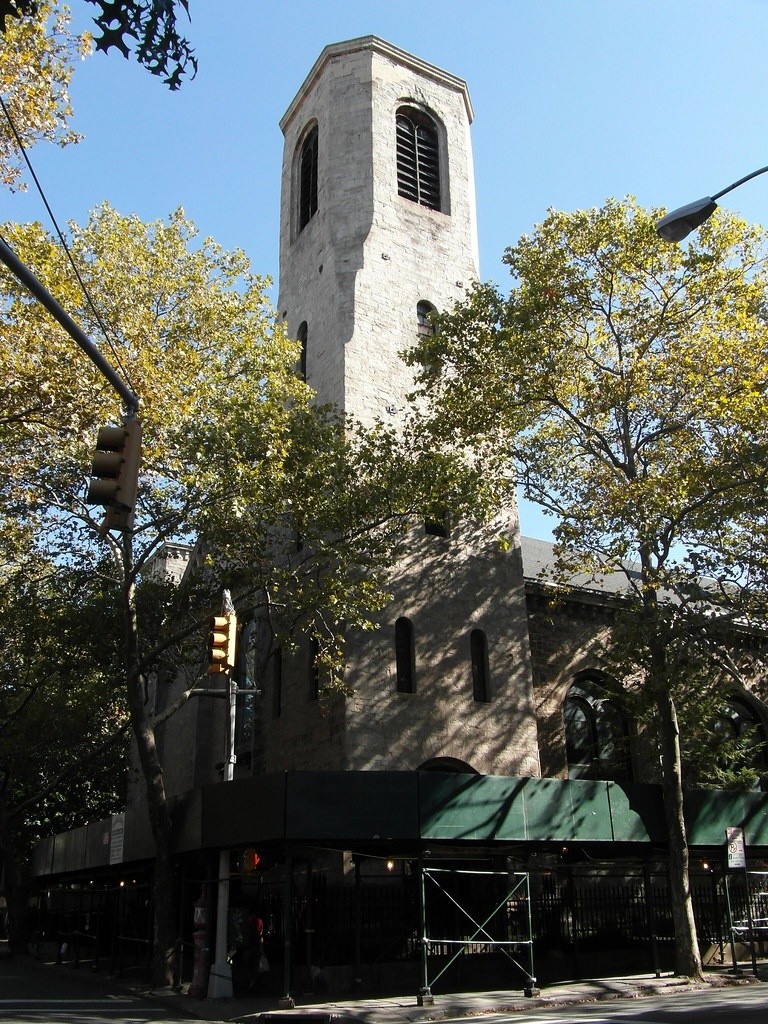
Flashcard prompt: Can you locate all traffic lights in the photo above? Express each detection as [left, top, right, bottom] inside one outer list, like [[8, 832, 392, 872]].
[[207, 613, 237, 674], [86, 417, 143, 533]]
